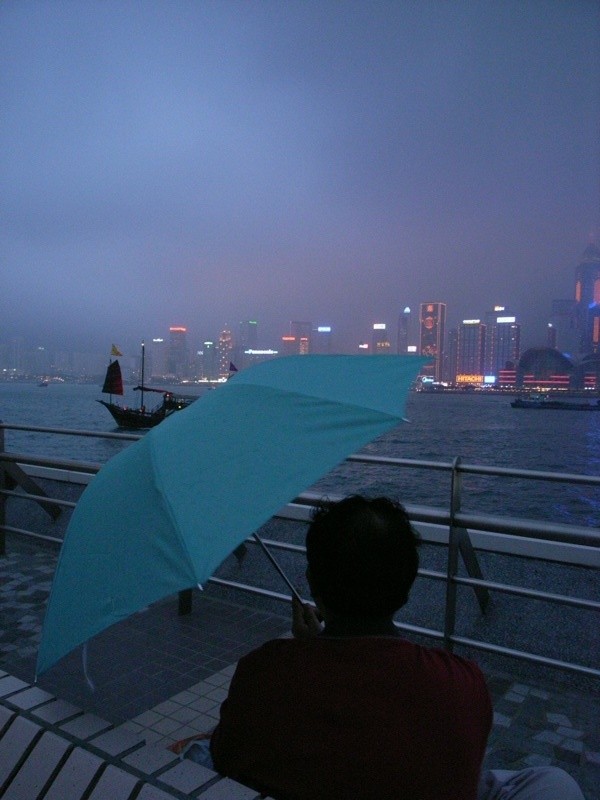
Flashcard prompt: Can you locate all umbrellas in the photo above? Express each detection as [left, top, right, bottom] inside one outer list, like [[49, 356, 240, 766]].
[[34, 353, 434, 680]]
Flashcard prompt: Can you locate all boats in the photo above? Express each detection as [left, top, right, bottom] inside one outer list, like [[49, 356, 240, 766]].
[[162, 361, 239, 411], [38, 382, 48, 388], [93, 339, 177, 431]]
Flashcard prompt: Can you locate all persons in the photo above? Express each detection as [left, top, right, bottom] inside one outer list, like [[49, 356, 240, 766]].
[[210, 493, 585, 800]]
